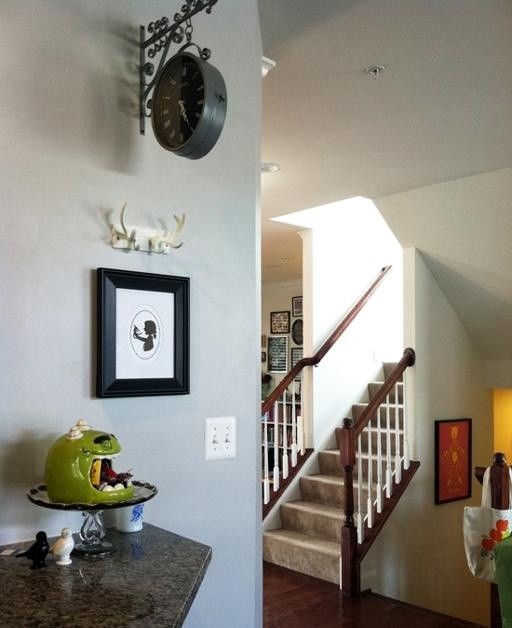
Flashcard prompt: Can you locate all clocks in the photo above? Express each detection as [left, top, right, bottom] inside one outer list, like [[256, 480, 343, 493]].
[[138, 0, 229, 162]]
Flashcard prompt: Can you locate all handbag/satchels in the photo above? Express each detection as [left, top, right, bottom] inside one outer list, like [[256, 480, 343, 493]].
[[463, 466, 511, 584]]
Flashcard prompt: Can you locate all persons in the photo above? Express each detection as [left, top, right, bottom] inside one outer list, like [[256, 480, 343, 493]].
[[131, 319, 158, 351]]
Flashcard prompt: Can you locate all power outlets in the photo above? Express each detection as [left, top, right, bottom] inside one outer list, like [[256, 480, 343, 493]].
[[204, 416, 237, 460]]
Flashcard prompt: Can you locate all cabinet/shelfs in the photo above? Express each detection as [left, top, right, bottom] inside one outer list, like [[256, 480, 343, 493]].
[[262, 399, 302, 450]]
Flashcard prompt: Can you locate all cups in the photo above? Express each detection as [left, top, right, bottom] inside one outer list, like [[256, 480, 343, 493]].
[[114, 500, 145, 533]]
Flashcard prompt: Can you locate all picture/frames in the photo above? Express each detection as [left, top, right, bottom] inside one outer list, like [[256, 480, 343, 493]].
[[265, 296, 303, 382], [434, 418, 472, 505], [95, 266, 191, 399]]
